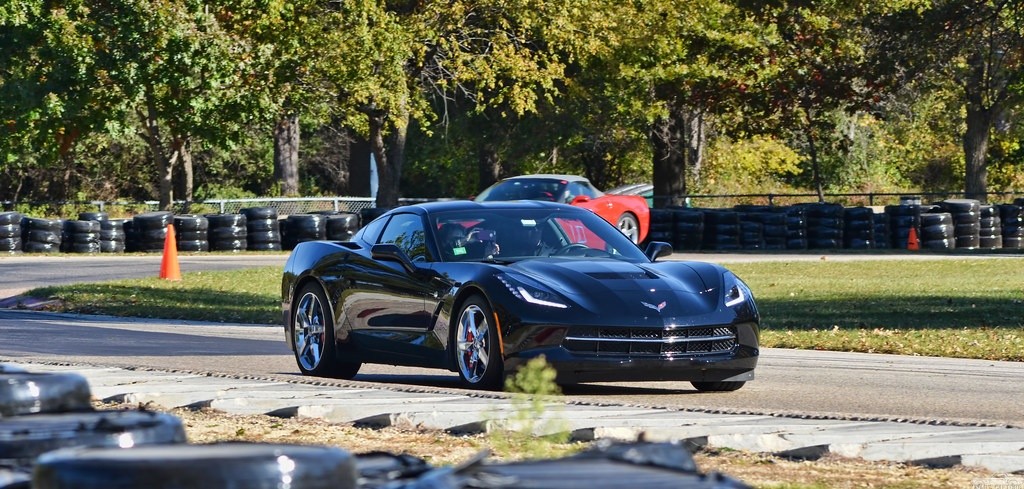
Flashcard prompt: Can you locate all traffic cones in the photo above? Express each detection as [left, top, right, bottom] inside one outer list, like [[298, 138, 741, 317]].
[[907, 227, 919, 250], [159, 224, 182, 281]]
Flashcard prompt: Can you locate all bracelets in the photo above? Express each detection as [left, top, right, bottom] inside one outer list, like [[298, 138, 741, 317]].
[[454, 239, 461, 248]]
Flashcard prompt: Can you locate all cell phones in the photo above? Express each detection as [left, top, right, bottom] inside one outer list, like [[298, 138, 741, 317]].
[[478, 230, 497, 241]]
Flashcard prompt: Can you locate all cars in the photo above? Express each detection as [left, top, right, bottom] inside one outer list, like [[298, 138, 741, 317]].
[[437, 175, 650, 257]]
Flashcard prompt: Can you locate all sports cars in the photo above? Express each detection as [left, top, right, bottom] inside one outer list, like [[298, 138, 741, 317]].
[[281, 201, 761, 392]]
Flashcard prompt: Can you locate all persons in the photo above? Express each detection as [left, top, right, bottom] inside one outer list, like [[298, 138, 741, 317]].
[[564, 189, 575, 204], [517, 228, 556, 256], [441, 224, 499, 258]]
